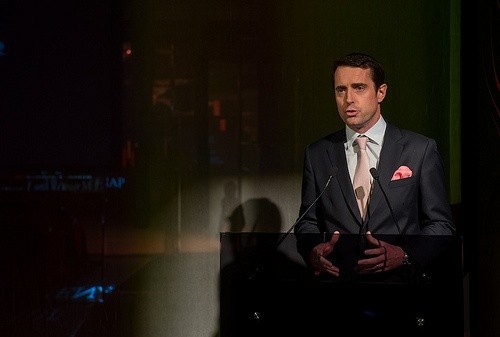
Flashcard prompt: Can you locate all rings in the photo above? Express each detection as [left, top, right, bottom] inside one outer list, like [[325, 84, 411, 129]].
[[376, 264, 379, 270]]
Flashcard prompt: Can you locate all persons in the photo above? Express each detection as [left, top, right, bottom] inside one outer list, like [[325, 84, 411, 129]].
[[294, 53, 458, 300]]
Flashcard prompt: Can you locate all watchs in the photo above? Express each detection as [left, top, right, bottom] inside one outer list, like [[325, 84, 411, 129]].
[[403, 247, 413, 265]]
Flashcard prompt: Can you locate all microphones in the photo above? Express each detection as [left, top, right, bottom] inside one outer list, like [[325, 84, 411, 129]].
[[274, 164, 339, 248], [370, 167, 401, 233]]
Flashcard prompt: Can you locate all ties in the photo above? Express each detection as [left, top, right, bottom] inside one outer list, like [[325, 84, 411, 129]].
[[352, 136, 371, 222]]
